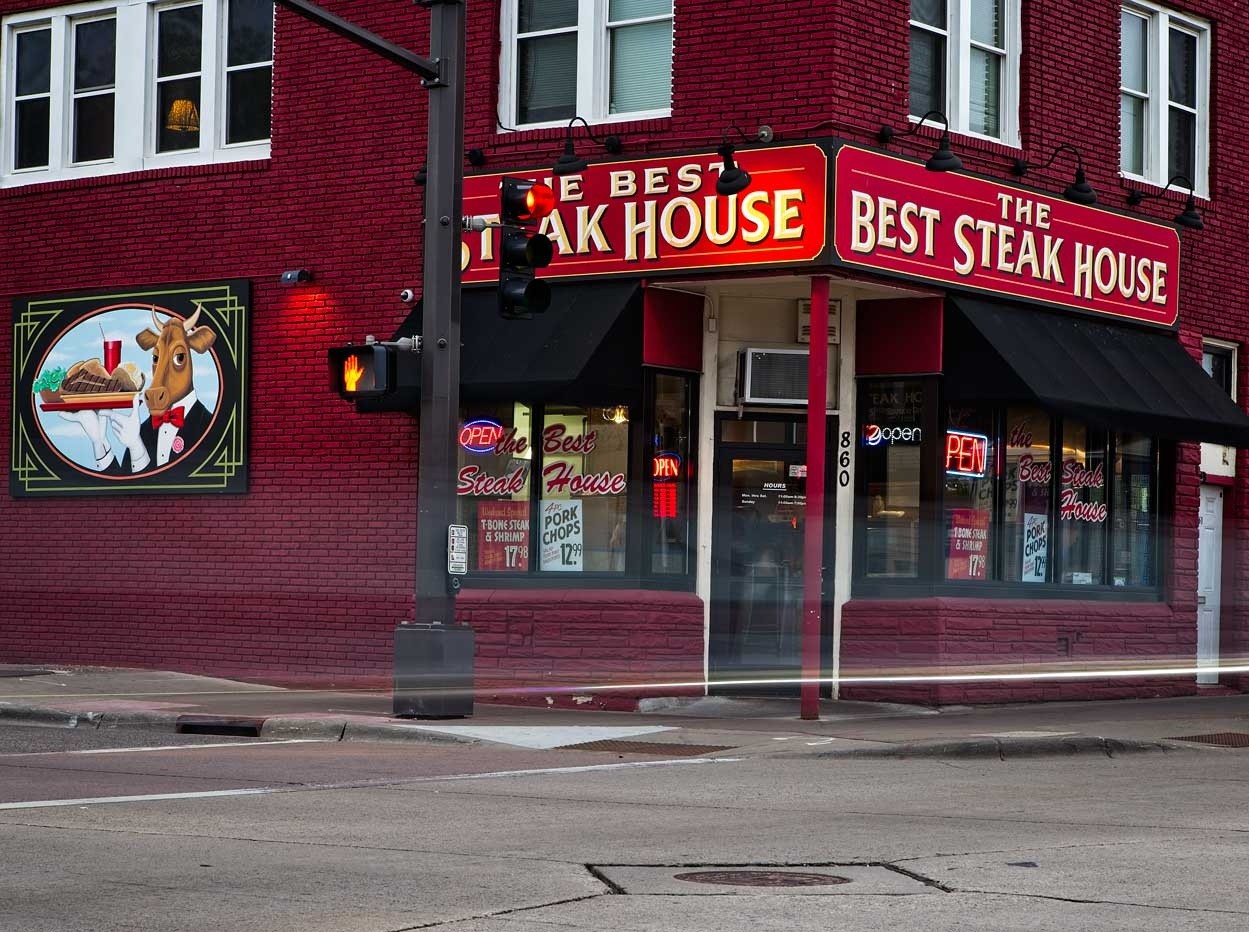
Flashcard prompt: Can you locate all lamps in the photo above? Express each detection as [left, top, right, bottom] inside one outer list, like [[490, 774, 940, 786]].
[[280, 269, 306, 282], [716, 126, 774, 196], [1011, 144, 1098, 205], [1126, 175, 1204, 231], [165, 99, 200, 150], [877, 110, 963, 172], [551, 116, 624, 177], [414, 147, 487, 185]]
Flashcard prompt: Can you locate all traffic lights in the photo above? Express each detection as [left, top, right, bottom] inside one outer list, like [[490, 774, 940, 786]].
[[494, 173, 554, 323], [327, 342, 397, 402]]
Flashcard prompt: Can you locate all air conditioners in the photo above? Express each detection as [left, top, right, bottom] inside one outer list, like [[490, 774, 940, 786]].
[[735, 345, 809, 406]]
